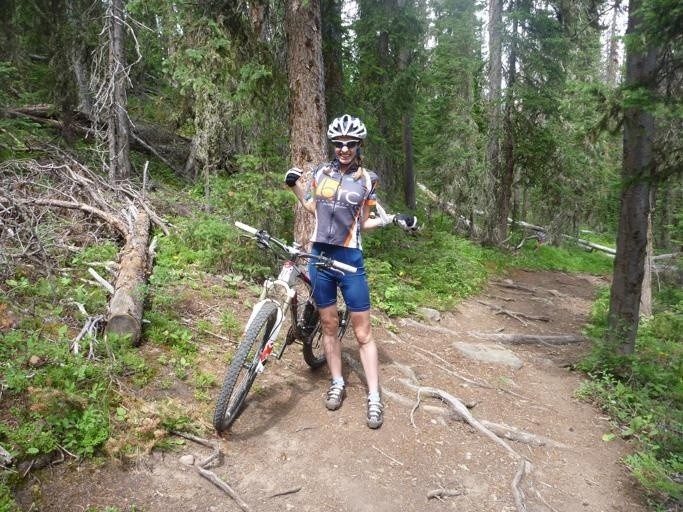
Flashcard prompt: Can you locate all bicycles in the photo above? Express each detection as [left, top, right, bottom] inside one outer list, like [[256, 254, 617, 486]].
[[212, 221, 358, 432]]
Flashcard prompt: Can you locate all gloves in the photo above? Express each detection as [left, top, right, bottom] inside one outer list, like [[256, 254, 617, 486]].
[[284, 166, 304, 187], [393, 212, 421, 233]]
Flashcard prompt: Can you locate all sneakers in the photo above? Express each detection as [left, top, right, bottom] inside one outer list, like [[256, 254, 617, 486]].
[[365, 393, 385, 429], [324, 380, 346, 411]]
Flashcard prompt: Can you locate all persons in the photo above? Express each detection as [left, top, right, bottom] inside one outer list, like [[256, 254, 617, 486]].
[[282, 114, 418, 429]]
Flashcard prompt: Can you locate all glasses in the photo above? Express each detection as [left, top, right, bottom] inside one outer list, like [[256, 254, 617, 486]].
[[330, 139, 361, 149]]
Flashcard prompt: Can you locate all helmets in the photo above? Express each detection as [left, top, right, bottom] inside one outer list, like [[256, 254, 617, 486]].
[[324, 114, 368, 142]]
